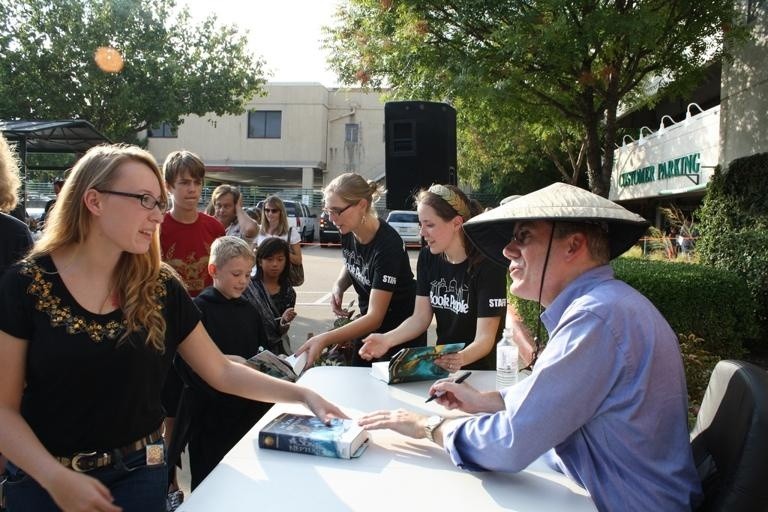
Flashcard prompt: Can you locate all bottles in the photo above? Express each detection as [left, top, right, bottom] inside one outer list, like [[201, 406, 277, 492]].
[[493, 327, 518, 393]]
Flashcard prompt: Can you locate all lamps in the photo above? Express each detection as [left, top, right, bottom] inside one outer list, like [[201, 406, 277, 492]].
[[622, 134, 636, 151], [639, 126, 654, 146], [657, 115, 676, 135], [685, 102, 716, 124]]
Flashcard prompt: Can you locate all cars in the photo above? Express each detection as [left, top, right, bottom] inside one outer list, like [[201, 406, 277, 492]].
[[319, 211, 343, 247], [26, 192, 57, 221]]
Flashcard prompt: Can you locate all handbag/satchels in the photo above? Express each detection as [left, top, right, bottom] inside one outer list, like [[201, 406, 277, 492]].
[[287, 250, 304, 287]]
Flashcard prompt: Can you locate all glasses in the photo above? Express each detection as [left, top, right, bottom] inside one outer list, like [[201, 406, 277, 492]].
[[264, 208, 279, 214], [97, 186, 168, 214], [322, 204, 354, 217]]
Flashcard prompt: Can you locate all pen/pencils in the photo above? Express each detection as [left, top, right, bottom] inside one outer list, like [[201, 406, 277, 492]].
[[425, 371, 472, 403]]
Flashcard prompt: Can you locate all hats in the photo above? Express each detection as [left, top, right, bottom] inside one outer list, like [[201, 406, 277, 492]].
[[460, 182, 650, 265]]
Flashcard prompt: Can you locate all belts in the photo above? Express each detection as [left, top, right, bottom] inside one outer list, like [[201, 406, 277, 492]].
[[56, 431, 161, 472]]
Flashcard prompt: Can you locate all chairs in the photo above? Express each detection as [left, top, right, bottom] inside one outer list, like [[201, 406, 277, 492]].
[[690, 359, 768, 512]]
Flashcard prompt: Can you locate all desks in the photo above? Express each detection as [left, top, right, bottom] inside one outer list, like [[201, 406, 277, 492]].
[[173, 365, 599, 512]]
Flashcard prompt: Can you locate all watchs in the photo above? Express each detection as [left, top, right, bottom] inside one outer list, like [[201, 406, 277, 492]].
[[425, 414, 446, 443]]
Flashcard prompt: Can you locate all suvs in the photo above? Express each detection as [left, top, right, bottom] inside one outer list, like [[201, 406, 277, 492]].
[[252, 200, 317, 248]]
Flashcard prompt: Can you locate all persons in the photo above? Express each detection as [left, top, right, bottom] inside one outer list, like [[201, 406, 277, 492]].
[[0, 133, 75, 272], [294, 172, 428, 366], [357, 182, 707, 512], [669, 220, 691, 252], [1, 142, 351, 512], [358, 185, 507, 371], [158, 150, 301, 511]]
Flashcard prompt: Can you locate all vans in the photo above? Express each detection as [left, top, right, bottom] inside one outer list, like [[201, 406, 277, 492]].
[[385, 210, 422, 248]]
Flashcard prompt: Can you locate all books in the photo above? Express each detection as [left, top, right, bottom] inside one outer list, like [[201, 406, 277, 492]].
[[247, 349, 307, 381], [259, 413, 366, 460], [370, 343, 466, 385]]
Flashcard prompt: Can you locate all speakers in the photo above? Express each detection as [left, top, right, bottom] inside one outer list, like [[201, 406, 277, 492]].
[[384, 100, 458, 211]]
